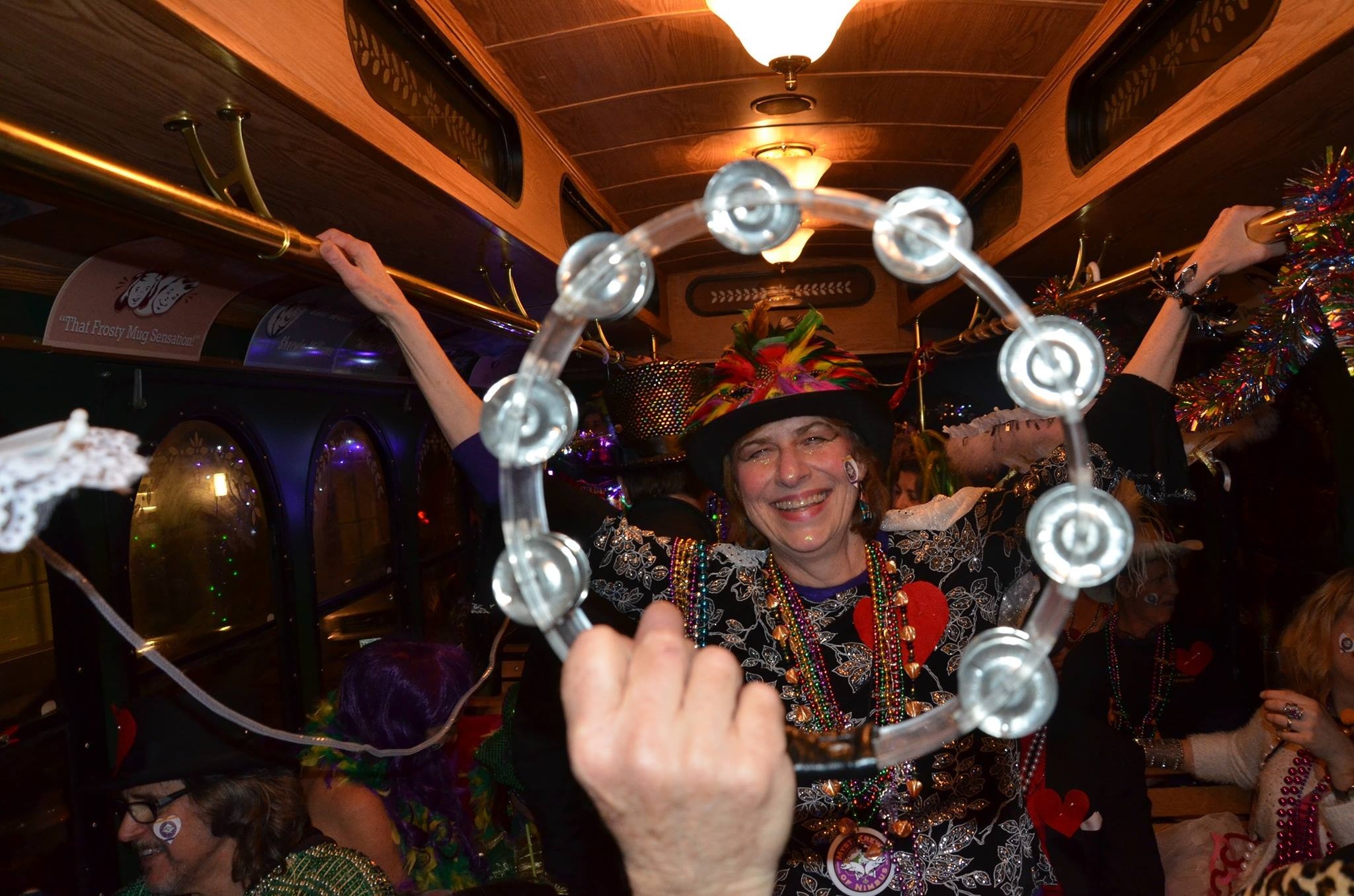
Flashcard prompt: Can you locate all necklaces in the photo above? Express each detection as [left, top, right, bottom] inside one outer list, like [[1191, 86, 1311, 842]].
[[1063, 599, 1104, 648], [1275, 744, 1331, 868], [1101, 609, 1177, 739], [658, 535, 924, 835]]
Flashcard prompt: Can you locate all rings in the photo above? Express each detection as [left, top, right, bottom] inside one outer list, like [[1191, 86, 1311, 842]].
[[1286, 717, 1293, 730], [1283, 701, 1304, 719]]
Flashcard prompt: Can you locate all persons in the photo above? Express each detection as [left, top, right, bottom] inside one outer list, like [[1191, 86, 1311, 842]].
[[111, 633, 395, 896], [1051, 579, 1120, 676], [576, 394, 614, 439], [298, 640, 556, 896], [883, 399, 987, 510], [559, 601, 797, 896], [311, 202, 1288, 896], [1058, 518, 1234, 784], [1132, 564, 1354, 896], [610, 349, 720, 546]]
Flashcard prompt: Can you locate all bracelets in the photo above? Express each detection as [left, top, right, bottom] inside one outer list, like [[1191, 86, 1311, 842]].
[[1134, 733, 1184, 772], [1147, 251, 1239, 339]]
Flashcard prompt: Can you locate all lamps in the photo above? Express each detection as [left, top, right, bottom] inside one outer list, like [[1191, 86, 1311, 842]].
[[706, 1, 862, 277]]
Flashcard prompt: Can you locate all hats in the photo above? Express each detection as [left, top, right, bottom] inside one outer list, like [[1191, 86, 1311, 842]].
[[1086, 524, 1205, 606], [676, 300, 896, 462], [105, 704, 249, 790]]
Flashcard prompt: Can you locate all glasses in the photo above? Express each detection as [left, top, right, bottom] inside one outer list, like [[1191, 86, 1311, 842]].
[[112, 784, 194, 823]]
